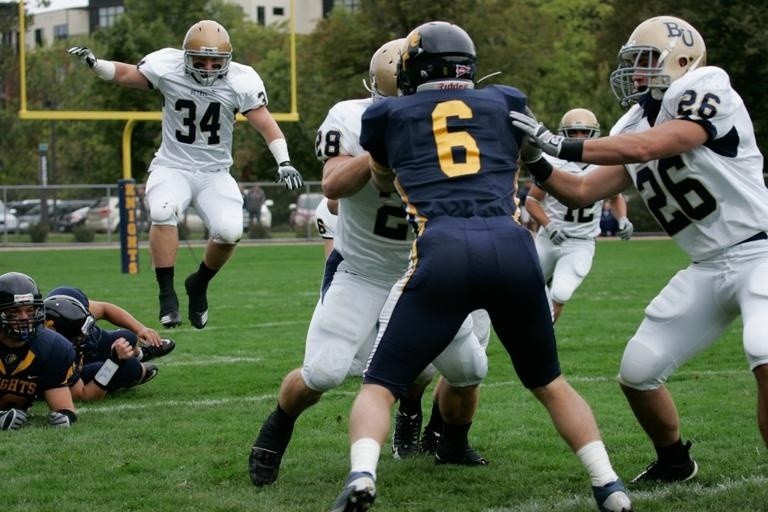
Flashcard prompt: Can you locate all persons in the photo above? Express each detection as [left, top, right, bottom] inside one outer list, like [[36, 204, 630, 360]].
[[525, 109, 634, 323], [519, 178, 538, 233], [510, 15, 767, 492], [330, 21, 630, 512], [0, 270, 79, 434], [40, 285, 178, 403], [64, 17, 306, 331], [248, 186, 265, 225], [599, 202, 617, 237], [249, 39, 489, 487]]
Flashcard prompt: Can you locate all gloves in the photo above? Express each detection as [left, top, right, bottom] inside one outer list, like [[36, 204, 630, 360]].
[[618, 215, 635, 241], [277, 160, 304, 190], [45, 409, 78, 426], [1, 408, 27, 430], [508, 105, 565, 166], [544, 219, 568, 244], [66, 42, 97, 69]]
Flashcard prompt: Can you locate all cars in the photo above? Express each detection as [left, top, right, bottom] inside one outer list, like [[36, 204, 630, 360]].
[[289, 191, 324, 234], [0, 187, 278, 237]]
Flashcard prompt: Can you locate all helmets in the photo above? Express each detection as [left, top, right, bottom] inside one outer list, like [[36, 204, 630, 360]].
[[368, 39, 407, 102], [1, 271, 47, 345], [182, 20, 232, 86], [394, 21, 478, 94], [43, 295, 104, 349], [611, 16, 707, 101], [557, 109, 601, 141]]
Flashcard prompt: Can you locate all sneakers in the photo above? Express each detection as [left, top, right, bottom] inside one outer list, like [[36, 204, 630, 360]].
[[392, 407, 489, 469], [326, 468, 377, 512], [250, 410, 289, 486], [591, 477, 633, 511], [138, 273, 208, 386], [630, 449, 698, 491]]
